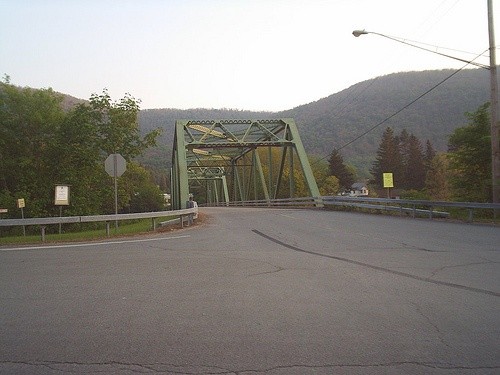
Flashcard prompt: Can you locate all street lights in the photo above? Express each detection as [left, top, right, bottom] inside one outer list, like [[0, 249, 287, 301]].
[[352, 28, 500, 221]]
